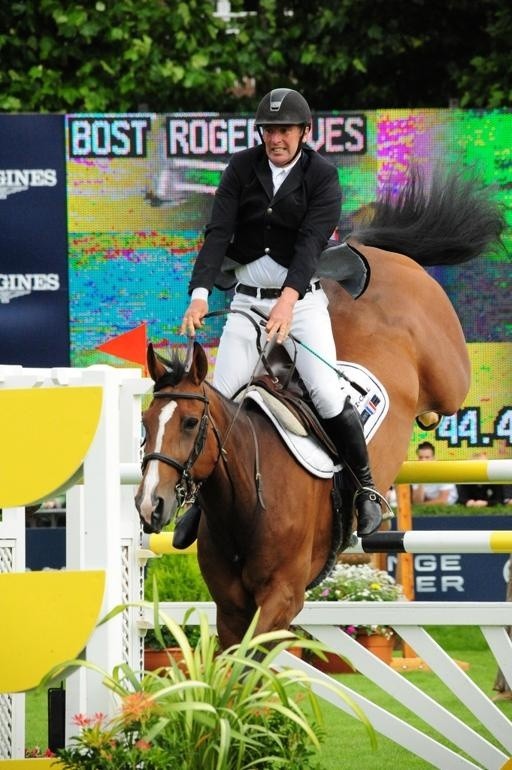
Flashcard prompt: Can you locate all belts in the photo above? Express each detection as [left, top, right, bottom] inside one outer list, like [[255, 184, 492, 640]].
[[236, 280, 322, 299]]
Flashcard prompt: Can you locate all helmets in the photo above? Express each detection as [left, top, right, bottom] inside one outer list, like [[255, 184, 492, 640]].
[[254, 87, 312, 127]]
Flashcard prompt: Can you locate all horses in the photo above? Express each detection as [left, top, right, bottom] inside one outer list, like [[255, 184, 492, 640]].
[[133, 148, 512, 707]]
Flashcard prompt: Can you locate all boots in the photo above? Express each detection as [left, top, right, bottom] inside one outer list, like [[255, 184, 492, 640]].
[[321, 395, 383, 536], [173, 497, 200, 548]]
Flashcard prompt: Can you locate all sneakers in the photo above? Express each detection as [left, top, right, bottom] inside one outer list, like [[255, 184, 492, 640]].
[[491, 690, 512, 700]]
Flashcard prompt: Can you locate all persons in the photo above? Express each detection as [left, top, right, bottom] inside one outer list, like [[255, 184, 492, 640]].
[[172, 86, 383, 548], [410, 440, 460, 509], [461, 450, 511, 509]]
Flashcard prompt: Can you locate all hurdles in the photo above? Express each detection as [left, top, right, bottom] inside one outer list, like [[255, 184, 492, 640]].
[[1, 364, 512, 770]]
